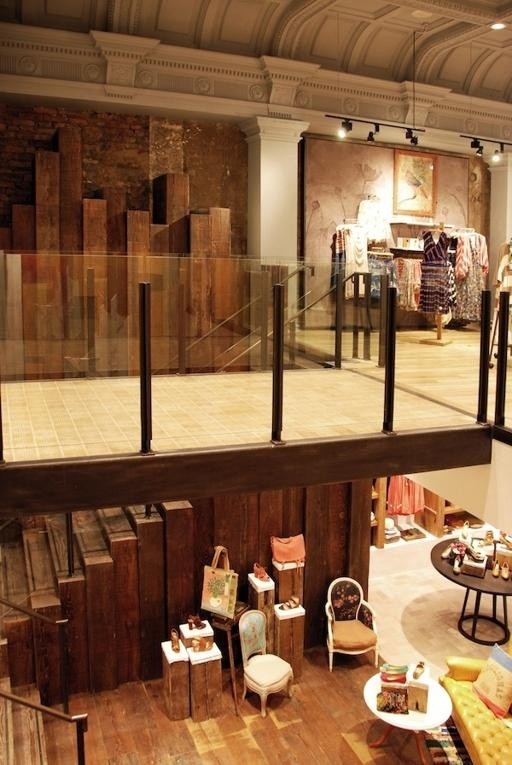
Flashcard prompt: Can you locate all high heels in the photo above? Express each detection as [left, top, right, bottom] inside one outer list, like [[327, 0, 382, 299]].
[[170, 615, 213, 653]]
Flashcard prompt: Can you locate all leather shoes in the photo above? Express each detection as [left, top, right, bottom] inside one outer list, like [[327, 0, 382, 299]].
[[380, 660, 424, 683], [441, 520, 512, 580]]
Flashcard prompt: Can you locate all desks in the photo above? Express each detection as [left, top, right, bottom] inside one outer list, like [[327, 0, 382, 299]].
[[361, 666, 456, 765], [209, 597, 252, 711], [428, 538, 512, 646]]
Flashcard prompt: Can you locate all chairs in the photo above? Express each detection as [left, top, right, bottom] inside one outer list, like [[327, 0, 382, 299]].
[[237, 608, 294, 718], [322, 576, 381, 672]]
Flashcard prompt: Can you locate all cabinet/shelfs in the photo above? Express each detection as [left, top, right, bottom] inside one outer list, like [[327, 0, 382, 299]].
[[414, 486, 484, 538], [387, 221, 455, 254], [369, 476, 387, 549]]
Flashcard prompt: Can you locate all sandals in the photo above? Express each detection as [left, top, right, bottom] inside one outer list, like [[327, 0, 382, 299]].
[[254, 562, 269, 582], [279, 595, 299, 611]]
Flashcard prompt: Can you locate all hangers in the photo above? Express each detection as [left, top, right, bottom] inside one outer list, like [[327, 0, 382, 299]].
[[426, 224, 444, 234], [449, 228, 480, 236]]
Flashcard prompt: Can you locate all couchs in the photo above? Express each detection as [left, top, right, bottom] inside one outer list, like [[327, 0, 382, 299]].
[[437, 653, 512, 765]]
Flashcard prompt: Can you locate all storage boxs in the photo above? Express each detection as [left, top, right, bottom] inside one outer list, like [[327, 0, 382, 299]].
[[461, 532, 512, 578]]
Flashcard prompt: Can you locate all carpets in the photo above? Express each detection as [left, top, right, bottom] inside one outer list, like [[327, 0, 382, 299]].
[[412, 716, 471, 765]]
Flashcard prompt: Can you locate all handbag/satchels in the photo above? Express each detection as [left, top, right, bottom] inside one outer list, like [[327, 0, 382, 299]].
[[200, 546, 240, 620], [271, 534, 306, 563]]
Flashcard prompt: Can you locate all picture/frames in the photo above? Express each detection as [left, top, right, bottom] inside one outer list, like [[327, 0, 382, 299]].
[[392, 147, 438, 218]]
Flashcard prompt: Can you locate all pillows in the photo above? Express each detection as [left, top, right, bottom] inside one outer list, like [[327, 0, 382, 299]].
[[471, 641, 512, 718]]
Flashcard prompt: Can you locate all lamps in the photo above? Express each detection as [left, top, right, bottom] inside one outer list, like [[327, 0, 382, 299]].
[[324, 110, 426, 149], [460, 131, 511, 165]]
